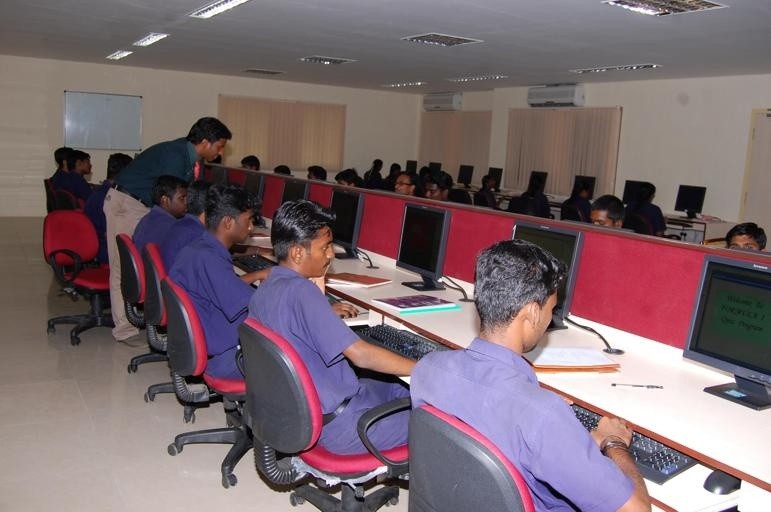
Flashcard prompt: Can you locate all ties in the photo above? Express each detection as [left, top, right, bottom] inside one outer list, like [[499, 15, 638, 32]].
[[193, 160, 201, 182]]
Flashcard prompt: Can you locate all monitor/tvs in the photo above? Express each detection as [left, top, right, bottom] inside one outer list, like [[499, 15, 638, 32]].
[[511, 220, 584, 334], [405, 160, 503, 193], [575, 175, 596, 200], [209, 167, 227, 184], [674, 184, 706, 219], [528, 170, 548, 193], [395, 202, 452, 292], [622, 180, 639, 204], [244, 172, 264, 200], [329, 187, 364, 260], [280, 181, 310, 204], [681, 253, 771, 409]]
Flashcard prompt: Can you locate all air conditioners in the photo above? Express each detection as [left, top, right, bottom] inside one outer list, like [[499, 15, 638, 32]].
[[527, 85, 584, 108], [424, 92, 462, 112]]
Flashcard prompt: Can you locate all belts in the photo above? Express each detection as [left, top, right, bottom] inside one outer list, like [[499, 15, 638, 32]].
[[110, 183, 146, 207], [321, 398, 353, 426]]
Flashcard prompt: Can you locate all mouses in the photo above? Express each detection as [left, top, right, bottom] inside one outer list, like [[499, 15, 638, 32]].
[[703, 469, 742, 495], [341, 312, 358, 318]]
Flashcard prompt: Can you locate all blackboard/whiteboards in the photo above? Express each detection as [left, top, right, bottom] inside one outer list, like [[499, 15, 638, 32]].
[[63, 90, 143, 151]]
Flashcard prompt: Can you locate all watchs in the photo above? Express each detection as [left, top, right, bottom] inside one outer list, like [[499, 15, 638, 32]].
[[601, 439, 629, 456]]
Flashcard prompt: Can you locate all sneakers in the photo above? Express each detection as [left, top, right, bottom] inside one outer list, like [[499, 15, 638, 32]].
[[119, 334, 149, 349]]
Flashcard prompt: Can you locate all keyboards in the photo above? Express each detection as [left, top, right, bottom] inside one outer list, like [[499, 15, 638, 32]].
[[325, 292, 340, 305], [668, 221, 693, 228], [232, 253, 278, 272], [568, 403, 698, 485], [354, 322, 439, 362]]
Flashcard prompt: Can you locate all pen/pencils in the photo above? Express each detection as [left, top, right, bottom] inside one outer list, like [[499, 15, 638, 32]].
[[611, 383, 664, 388]]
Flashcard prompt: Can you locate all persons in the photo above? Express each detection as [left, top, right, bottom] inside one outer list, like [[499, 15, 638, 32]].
[[409, 239, 651, 512], [102, 117, 233, 350], [49, 146, 74, 189], [248, 197, 417, 456], [239, 155, 681, 241], [60, 149, 93, 201], [160, 179, 214, 276], [725, 221, 768, 254], [83, 154, 135, 265], [209, 154, 222, 164], [133, 173, 189, 258], [166, 181, 272, 381]]
